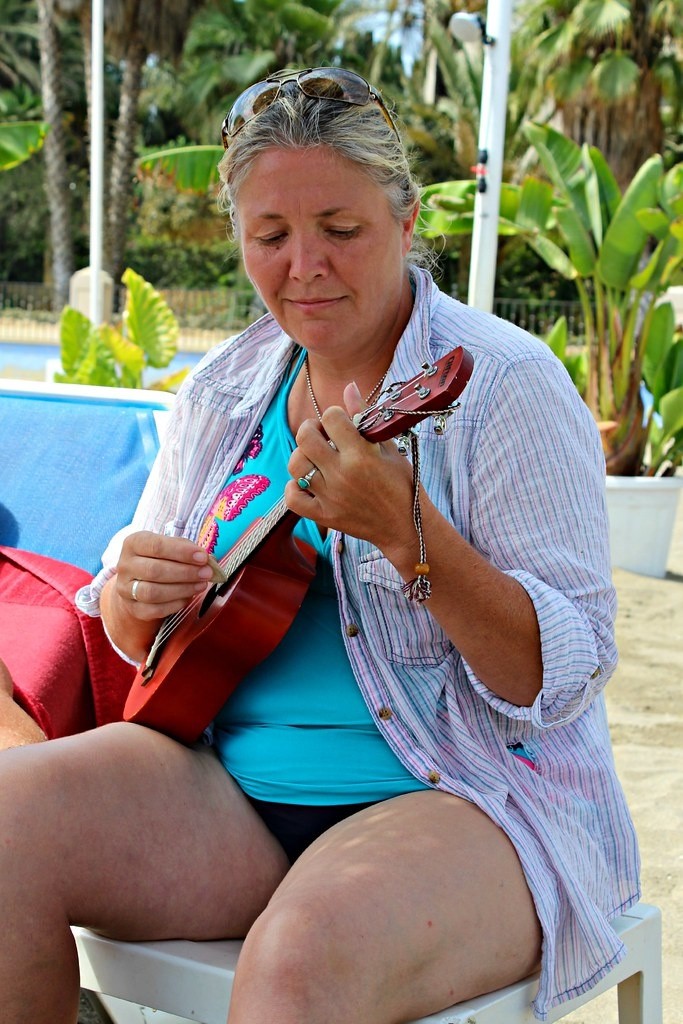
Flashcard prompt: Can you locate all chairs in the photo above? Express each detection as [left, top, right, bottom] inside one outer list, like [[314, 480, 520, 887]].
[[2, 378, 176, 579]]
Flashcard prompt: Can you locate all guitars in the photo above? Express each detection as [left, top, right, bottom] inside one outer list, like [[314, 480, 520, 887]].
[[123, 344, 476, 740]]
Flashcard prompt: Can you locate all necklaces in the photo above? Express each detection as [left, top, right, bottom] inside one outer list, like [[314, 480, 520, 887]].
[[304, 356, 390, 422]]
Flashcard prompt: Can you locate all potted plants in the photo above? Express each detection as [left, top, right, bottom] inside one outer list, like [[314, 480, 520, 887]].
[[407, 119, 682, 580]]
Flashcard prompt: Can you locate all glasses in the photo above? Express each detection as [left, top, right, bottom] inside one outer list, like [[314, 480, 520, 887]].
[[221, 66, 401, 152]]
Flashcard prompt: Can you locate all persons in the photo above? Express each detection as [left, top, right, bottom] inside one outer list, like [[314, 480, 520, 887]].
[[0, 65, 641, 1024]]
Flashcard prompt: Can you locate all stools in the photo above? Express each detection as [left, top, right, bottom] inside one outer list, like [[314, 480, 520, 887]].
[[68, 904, 664, 1024]]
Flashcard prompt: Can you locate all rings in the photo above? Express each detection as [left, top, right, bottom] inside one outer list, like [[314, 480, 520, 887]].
[[297, 467, 317, 489], [130, 580, 141, 601]]
